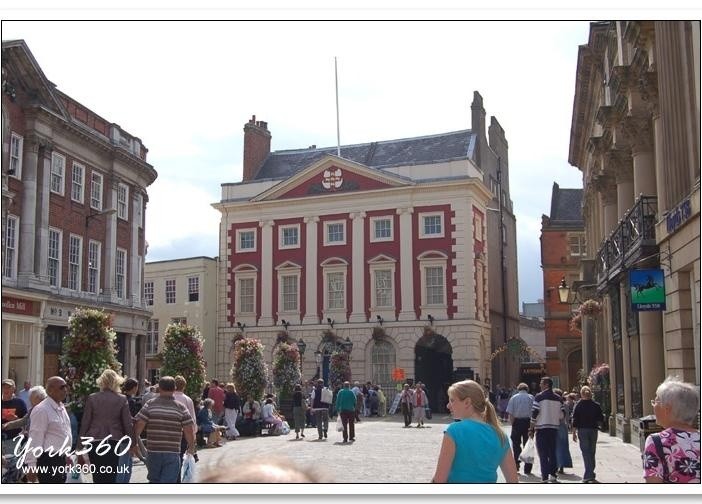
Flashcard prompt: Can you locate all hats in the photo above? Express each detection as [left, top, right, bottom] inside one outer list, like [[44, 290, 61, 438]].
[[1, 379, 14, 386]]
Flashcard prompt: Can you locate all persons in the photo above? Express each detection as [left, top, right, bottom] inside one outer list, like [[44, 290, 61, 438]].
[[2, 367, 700, 484]]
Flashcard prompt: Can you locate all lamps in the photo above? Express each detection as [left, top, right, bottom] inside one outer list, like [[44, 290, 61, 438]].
[[85, 208, 117, 227], [557, 276, 570, 304]]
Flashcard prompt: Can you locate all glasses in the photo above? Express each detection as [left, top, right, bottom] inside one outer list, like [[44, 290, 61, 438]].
[[651, 399, 661, 406]]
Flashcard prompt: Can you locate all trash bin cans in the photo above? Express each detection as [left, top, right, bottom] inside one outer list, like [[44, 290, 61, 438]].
[[639, 414, 663, 452]]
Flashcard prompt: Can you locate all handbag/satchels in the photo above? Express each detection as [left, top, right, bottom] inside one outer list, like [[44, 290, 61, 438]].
[[320, 388, 333, 404]]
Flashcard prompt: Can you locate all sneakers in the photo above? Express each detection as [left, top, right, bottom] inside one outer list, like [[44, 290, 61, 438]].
[[195, 434, 356, 448], [362, 414, 426, 428], [542, 470, 598, 483]]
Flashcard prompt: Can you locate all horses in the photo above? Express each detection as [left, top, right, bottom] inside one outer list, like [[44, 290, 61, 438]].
[[634, 275, 662, 297]]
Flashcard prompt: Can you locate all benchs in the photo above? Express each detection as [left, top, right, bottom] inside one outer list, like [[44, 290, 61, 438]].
[[200, 427, 230, 447], [260, 421, 273, 436]]
